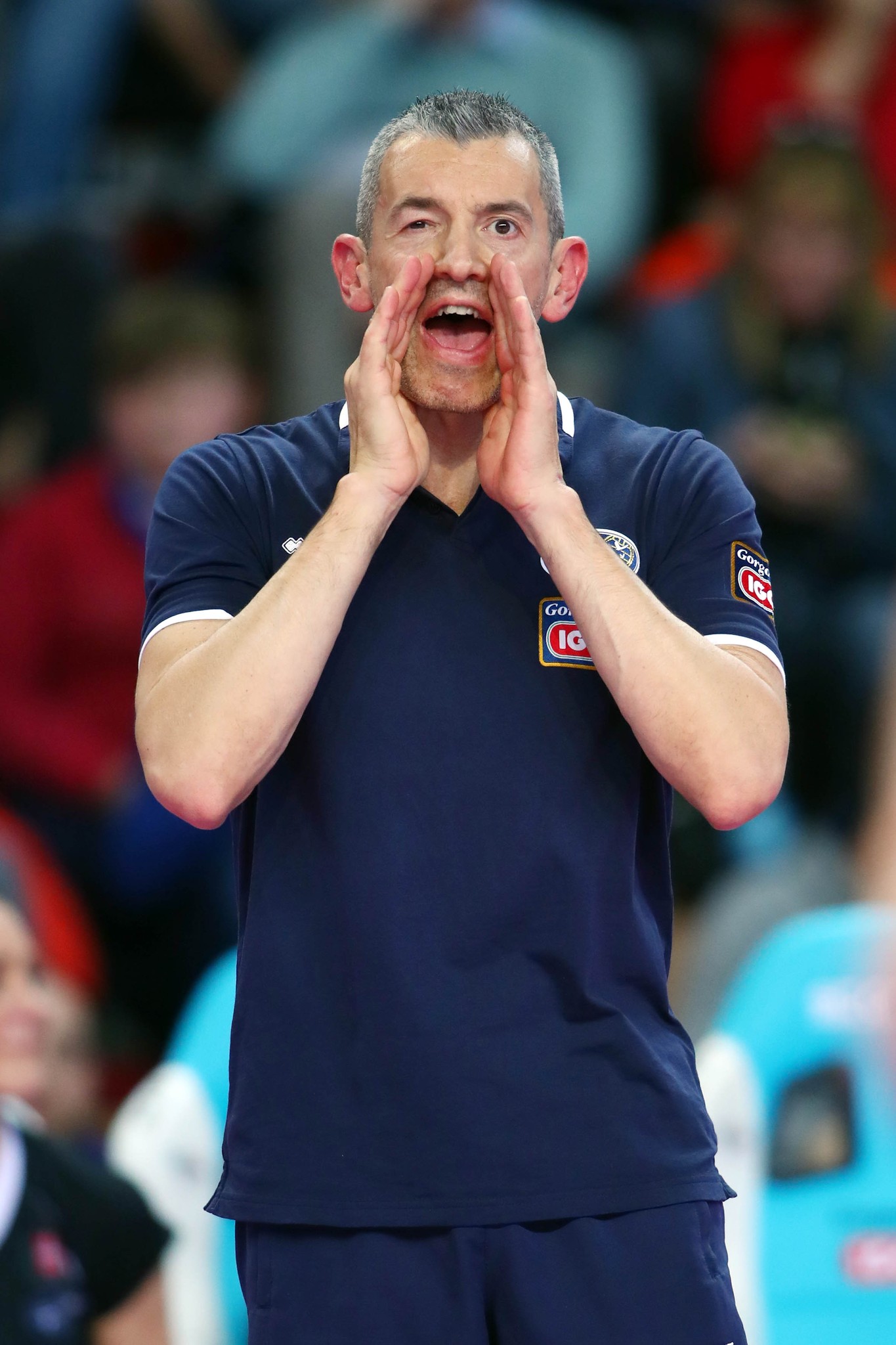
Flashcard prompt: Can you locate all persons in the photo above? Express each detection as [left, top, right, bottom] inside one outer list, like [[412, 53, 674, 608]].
[[133, 86, 791, 1345], [0, 0, 896, 1345]]
